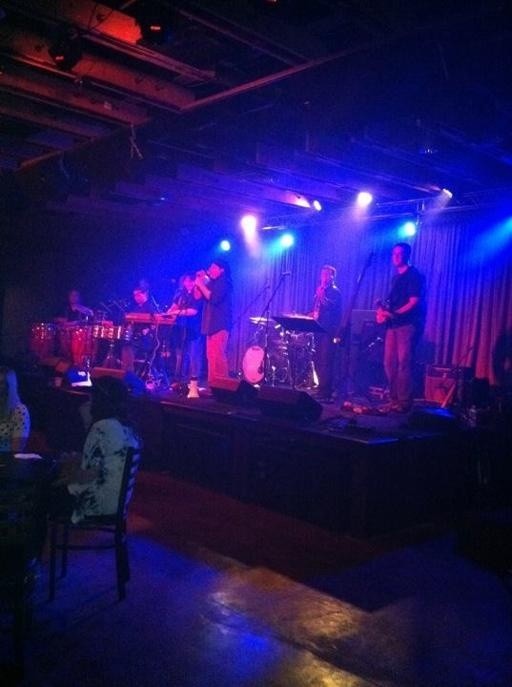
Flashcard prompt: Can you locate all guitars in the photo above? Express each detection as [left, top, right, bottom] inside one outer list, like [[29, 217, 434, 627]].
[[375, 298, 393, 325]]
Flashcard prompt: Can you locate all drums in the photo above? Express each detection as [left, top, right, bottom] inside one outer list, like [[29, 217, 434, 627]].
[[69, 326, 98, 368], [241, 345, 289, 384], [92, 324, 117, 340], [28, 322, 56, 354], [60, 322, 78, 357]]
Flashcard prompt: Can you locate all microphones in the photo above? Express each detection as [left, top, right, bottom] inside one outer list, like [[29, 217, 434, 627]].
[[281, 272, 292, 277], [201, 277, 211, 286]]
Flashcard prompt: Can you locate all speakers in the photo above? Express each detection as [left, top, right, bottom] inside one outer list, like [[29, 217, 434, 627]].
[[91, 367, 147, 398], [209, 376, 257, 407], [257, 384, 323, 421]]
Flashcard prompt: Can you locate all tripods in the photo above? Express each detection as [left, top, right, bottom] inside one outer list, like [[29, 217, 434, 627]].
[[327, 259, 374, 404]]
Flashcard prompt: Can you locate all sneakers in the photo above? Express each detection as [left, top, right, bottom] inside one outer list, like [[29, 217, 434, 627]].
[[378, 401, 407, 414]]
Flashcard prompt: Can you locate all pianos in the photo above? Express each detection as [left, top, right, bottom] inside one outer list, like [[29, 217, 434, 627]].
[[124, 312, 190, 326]]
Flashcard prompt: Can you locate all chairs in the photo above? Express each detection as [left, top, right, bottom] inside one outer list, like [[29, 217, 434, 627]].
[[47, 440, 142, 604]]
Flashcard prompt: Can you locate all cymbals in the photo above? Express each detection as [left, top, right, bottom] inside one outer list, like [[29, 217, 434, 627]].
[[72, 304, 93, 317], [250, 317, 272, 322]]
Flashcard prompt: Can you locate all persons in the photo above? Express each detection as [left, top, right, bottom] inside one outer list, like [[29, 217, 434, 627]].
[[0, 361, 31, 453], [306, 264, 343, 401], [56, 258, 233, 403], [25, 373, 141, 603], [373, 241, 421, 416]]
